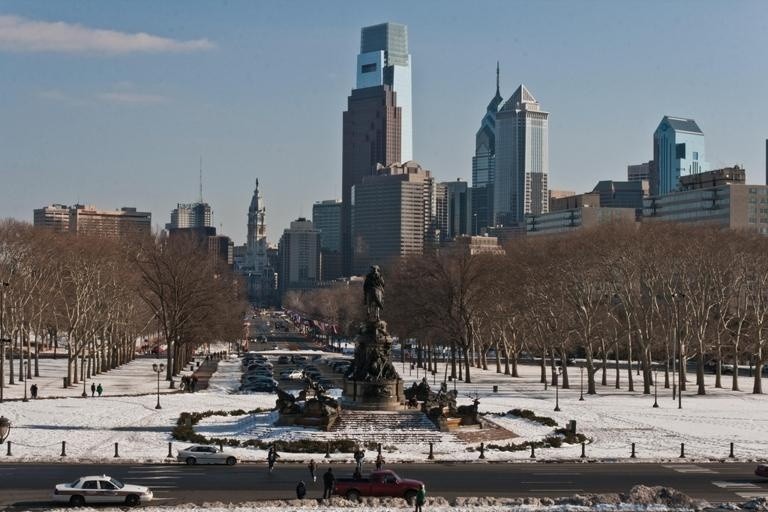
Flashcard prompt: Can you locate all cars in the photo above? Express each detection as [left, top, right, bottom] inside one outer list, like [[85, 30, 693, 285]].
[[51, 473, 154, 507], [176, 445, 242, 466], [238, 351, 355, 394]]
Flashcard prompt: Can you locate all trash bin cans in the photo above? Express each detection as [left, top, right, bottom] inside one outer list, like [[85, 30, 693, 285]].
[[64, 377, 68, 388]]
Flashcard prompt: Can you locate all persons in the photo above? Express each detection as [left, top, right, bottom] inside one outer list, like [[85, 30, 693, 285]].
[[182, 374, 198, 393], [206, 350, 227, 362], [414, 484, 425, 512], [362, 264, 385, 306], [352, 449, 365, 481], [268, 447, 281, 472], [30, 384, 38, 398], [91, 383, 103, 396], [323, 467, 336, 499], [308, 459, 319, 483], [296, 479, 306, 499], [376, 451, 385, 471]]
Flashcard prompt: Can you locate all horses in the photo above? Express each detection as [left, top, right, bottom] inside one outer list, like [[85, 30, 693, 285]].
[[366, 273, 385, 324]]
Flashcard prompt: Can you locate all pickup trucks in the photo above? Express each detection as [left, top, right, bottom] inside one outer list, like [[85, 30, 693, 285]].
[[331, 468, 426, 506]]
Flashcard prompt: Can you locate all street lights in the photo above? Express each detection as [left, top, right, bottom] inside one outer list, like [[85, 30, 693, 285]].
[[551, 367, 563, 412], [578, 360, 586, 401], [151, 363, 165, 409], [22, 360, 30, 402], [81, 359, 88, 397], [652, 366, 660, 408]]
